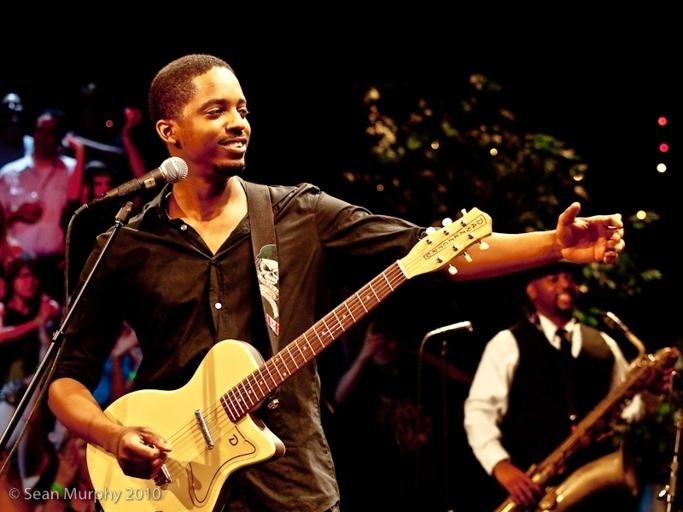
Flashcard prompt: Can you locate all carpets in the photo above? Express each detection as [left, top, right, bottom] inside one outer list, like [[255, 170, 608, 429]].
[[555, 328, 572, 354]]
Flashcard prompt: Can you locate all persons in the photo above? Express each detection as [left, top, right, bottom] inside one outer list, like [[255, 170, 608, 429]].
[[0, 90, 150, 512], [333, 321, 410, 406], [461, 258, 681, 512], [45, 52, 625, 511]]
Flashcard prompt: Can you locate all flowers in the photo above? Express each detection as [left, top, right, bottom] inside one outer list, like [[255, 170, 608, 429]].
[[85, 157, 189, 211]]
